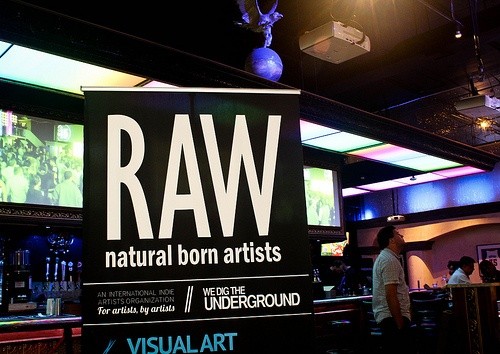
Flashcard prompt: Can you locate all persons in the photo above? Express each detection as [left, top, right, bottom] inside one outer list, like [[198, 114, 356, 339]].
[[321, 259, 348, 288], [448, 256, 475, 284], [306, 193, 335, 226], [0, 142, 83, 207], [372, 226, 411, 354], [479, 259, 500, 282]]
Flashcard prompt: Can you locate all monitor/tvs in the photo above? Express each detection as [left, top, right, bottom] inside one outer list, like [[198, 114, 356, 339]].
[[302, 162, 343, 230], [0, 107, 83, 211]]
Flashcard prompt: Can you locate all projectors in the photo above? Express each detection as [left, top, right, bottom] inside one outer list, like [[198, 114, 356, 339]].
[[298, 21, 370, 64]]
